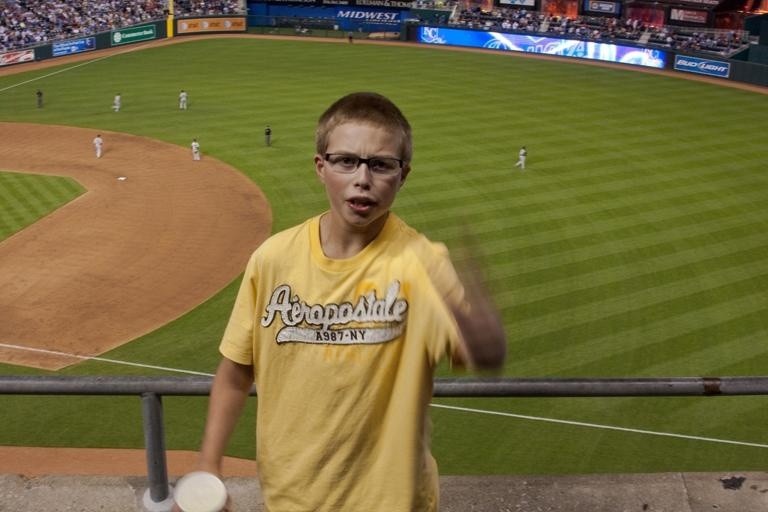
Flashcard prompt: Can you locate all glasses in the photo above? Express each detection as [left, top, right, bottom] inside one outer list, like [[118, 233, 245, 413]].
[[324, 152, 404, 176]]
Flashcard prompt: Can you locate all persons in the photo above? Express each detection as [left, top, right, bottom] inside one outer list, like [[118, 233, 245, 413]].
[[0, 0, 166, 55], [108, 92, 122, 114], [263, 125, 271, 147], [36, 88, 45, 108], [189, 137, 202, 162], [412, 0, 460, 10], [646, 24, 741, 54], [512, 146, 528, 170], [91, 133, 104, 159], [348, 29, 353, 45], [452, 5, 642, 43], [165, 1, 249, 19], [163, 90, 509, 512], [177, 89, 190, 111]]
